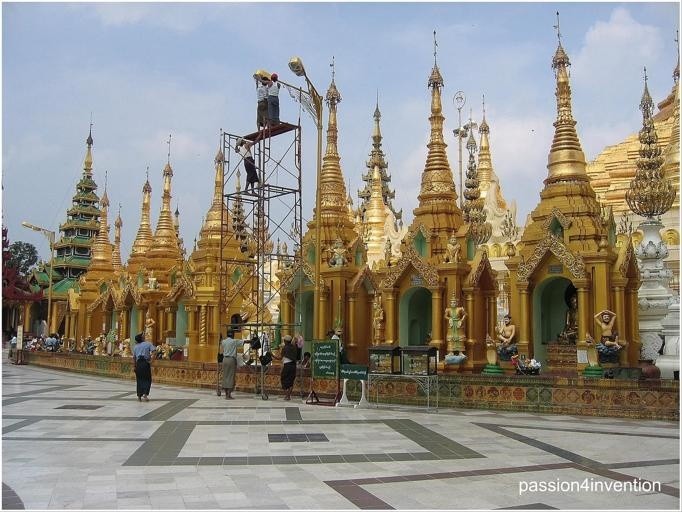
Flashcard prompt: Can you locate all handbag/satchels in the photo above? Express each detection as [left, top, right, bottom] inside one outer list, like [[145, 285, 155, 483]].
[[259, 352, 273, 366], [250, 333, 261, 349]]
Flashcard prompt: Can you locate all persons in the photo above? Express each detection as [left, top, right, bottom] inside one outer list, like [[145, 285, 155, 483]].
[[236, 133, 262, 195], [496, 315, 516, 350], [278, 334, 298, 400], [143, 312, 155, 336], [557, 297, 578, 340], [222, 330, 252, 400], [444, 294, 468, 356], [131, 332, 160, 401], [333, 243, 348, 266], [371, 301, 384, 348], [299, 352, 311, 368], [254, 73, 280, 131], [444, 238, 463, 263], [594, 309, 621, 350], [326, 329, 344, 363], [9, 333, 63, 351]]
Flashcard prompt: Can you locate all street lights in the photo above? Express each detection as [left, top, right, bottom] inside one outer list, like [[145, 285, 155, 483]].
[[253, 56, 325, 341], [22, 221, 56, 336]]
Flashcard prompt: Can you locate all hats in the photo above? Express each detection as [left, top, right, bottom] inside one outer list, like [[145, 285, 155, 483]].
[[135, 331, 143, 341], [281, 334, 291, 341], [271, 73, 278, 78], [236, 136, 244, 145], [226, 329, 234, 335]]
